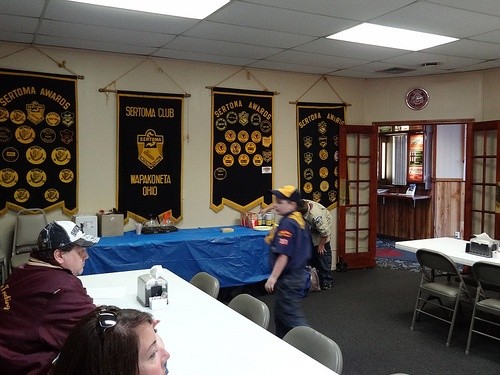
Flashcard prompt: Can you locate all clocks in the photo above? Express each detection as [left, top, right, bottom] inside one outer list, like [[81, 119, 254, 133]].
[[405, 87, 430, 110]]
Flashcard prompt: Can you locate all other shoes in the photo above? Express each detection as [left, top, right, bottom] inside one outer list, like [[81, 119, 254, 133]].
[[323, 281, 332, 289]]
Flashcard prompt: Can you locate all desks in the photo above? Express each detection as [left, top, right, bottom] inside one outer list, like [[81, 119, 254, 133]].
[[77, 224, 280, 289], [378, 192, 430, 208], [395, 236, 500, 321], [76, 268, 342, 375]]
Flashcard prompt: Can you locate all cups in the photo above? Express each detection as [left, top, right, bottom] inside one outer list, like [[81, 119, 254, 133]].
[[135, 224, 143, 235], [455, 231, 461, 240]]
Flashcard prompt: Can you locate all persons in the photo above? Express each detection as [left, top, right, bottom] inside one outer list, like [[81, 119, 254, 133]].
[[264, 184, 312, 340], [297, 198, 335, 291], [0, 220, 98, 375], [48, 304, 171, 375]]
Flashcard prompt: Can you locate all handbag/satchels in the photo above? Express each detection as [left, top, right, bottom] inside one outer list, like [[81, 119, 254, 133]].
[[309, 266, 321, 292]]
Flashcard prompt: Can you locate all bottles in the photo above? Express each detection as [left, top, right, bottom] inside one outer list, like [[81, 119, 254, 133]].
[[241, 210, 272, 226], [144, 214, 172, 226]]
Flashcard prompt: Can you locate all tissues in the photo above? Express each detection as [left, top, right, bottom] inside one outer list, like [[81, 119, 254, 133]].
[[136, 265, 169, 308], [469, 232, 499, 258]]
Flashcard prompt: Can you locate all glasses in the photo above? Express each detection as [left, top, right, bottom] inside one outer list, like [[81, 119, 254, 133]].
[[98, 306, 120, 347]]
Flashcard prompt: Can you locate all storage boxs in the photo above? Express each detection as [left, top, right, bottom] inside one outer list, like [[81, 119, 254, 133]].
[[470, 242, 497, 257], [97, 214, 123, 237], [72, 215, 99, 238]]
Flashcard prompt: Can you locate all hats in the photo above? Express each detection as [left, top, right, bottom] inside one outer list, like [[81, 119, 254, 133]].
[[37, 220, 100, 251], [271, 185, 300, 203]]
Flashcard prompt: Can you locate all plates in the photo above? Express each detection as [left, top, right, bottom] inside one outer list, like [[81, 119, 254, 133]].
[[253, 225, 273, 231]]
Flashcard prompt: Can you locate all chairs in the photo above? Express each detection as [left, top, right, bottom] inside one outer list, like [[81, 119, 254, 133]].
[[411, 249, 500, 354], [188, 271, 219, 298], [228, 294, 272, 328], [282, 325, 344, 375]]
